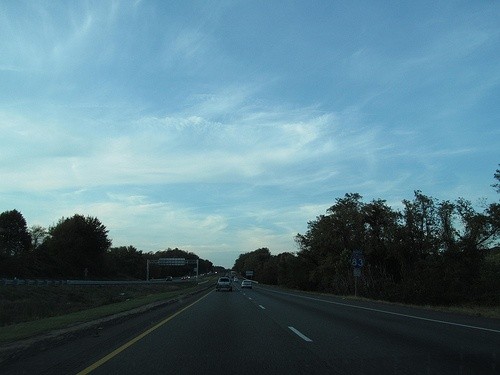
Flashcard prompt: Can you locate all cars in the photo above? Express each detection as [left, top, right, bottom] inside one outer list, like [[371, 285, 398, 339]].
[[240, 280, 252, 289], [233, 278, 239, 283], [165, 275, 173, 281], [180, 273, 197, 280], [216, 276, 233, 291]]
